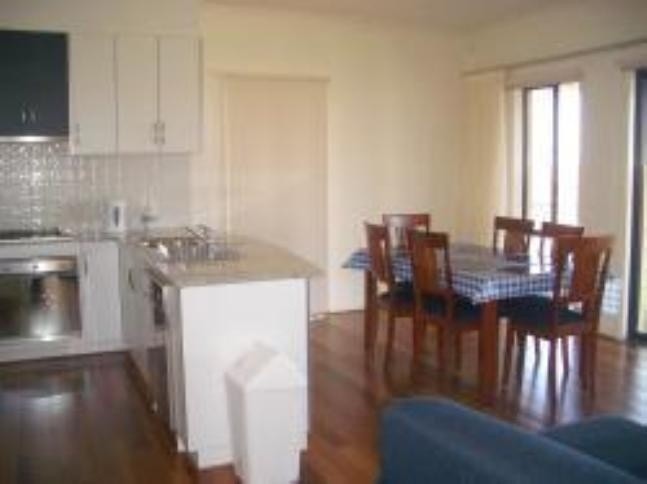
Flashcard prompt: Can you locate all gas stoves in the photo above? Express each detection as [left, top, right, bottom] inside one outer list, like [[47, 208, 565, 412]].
[[0, 226, 75, 244]]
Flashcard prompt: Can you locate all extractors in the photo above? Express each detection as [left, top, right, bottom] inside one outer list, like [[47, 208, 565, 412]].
[[0, 30, 68, 152]]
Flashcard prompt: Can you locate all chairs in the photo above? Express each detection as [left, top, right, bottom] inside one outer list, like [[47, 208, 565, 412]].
[[503, 222, 586, 386], [382, 213, 430, 251], [494, 216, 535, 254], [405, 226, 482, 393], [502, 235, 618, 401], [363, 220, 414, 375]]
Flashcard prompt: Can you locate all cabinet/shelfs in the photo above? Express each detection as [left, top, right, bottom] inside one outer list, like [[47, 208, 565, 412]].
[[67, 35, 116, 155], [114, 36, 200, 154], [122, 244, 149, 377], [77, 241, 121, 342]]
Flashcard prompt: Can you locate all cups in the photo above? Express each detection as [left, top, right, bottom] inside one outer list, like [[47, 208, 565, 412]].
[[170, 236, 240, 272]]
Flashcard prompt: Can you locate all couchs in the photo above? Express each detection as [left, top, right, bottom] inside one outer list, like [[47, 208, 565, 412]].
[[378, 396, 647, 484]]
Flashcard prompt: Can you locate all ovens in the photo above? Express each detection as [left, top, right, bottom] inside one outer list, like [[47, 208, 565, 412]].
[[1, 255, 83, 346]]
